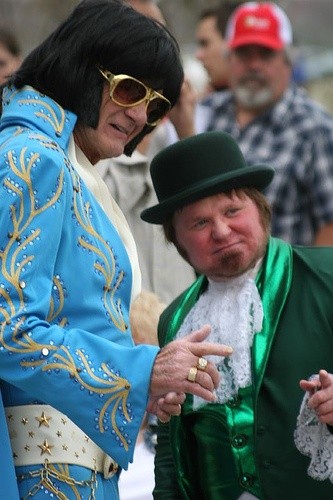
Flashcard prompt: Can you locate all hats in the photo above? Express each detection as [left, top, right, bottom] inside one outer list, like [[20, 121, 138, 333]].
[[139, 130, 276, 224], [224, 0, 294, 54]]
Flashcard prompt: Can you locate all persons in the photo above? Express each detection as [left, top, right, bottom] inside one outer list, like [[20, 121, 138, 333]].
[[0, 1, 233, 500], [93, 0, 245, 305], [0, 26, 23, 87], [139, 130, 333, 500], [196, 0, 333, 247]]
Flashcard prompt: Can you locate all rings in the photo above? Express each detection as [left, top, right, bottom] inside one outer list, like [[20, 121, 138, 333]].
[[196, 357, 207, 370], [186, 367, 197, 382]]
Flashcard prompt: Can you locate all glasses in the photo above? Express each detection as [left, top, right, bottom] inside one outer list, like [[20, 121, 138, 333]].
[[91, 58, 172, 128]]
